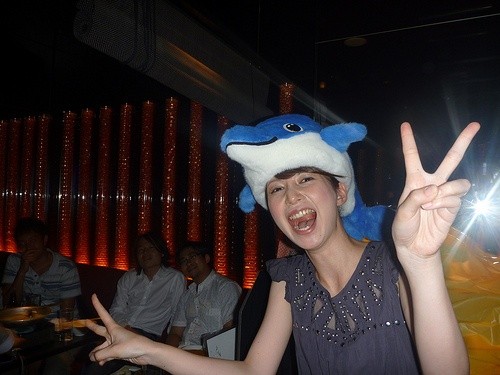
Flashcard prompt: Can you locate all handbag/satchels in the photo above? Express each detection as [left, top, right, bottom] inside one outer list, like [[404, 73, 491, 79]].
[[440, 227, 500, 375]]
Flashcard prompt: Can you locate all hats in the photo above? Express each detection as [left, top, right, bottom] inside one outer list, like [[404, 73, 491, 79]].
[[220, 113, 395, 242]]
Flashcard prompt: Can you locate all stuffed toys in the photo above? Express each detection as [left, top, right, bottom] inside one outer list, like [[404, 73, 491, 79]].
[[222, 114, 394, 243]]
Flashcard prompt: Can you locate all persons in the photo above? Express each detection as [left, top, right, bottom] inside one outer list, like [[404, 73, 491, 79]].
[[84, 122, 482, 375], [86, 231, 187, 375], [166, 241, 241, 354], [2, 217, 81, 319]]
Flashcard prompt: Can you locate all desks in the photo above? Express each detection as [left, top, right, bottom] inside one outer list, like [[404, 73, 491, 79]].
[[0, 320, 105, 375]]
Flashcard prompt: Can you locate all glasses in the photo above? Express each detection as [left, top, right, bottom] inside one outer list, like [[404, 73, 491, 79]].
[[180, 253, 200, 264]]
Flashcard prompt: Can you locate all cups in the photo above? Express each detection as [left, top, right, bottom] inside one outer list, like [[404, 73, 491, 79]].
[[59, 308, 75, 341]]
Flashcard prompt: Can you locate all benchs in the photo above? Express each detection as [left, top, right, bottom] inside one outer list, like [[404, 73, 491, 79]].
[[0, 252, 246, 337]]
